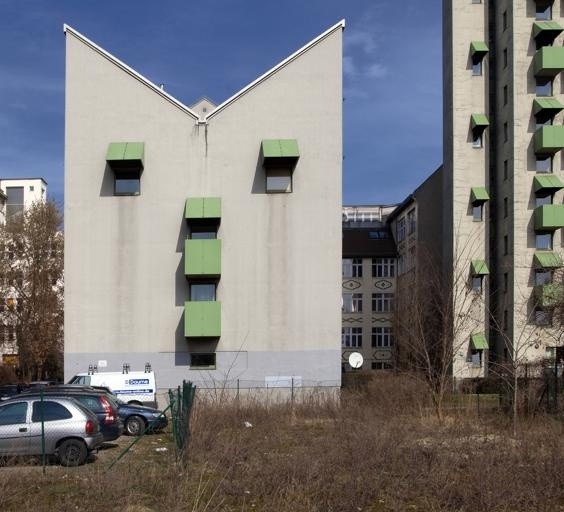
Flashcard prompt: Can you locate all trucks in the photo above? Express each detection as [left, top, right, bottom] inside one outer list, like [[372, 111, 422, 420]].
[[65, 362, 159, 414]]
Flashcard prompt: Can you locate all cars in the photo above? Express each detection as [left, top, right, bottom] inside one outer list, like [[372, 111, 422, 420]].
[[0, 397, 104, 470], [87, 383, 168, 438]]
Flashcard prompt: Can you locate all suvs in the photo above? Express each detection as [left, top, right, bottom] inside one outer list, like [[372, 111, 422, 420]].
[[3, 386, 126, 447]]
